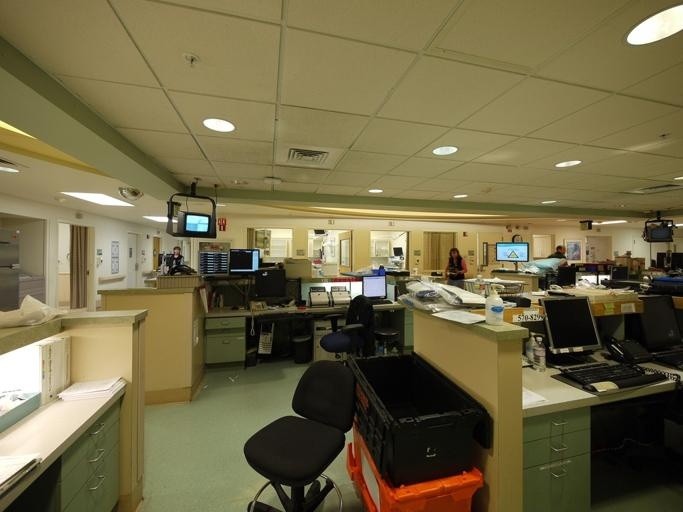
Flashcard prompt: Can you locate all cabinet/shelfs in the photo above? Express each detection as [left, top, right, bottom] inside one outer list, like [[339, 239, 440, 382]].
[[532, 233, 555, 259]]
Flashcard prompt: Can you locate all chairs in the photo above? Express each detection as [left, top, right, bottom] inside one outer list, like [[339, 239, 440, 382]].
[[320, 294, 374, 368], [244, 359, 359, 512]]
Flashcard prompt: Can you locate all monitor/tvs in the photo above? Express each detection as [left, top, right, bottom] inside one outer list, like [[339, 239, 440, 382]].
[[647, 226, 673, 241], [177, 212, 212, 237], [656, 252, 683, 271], [254, 270, 288, 304], [557, 266, 576, 288], [392, 247, 403, 257], [362, 275, 387, 300], [611, 266, 629, 285], [540, 295, 602, 365], [626, 295, 683, 355], [230, 248, 260, 278], [496, 242, 530, 273]]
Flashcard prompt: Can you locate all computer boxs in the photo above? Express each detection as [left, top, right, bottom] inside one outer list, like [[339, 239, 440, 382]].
[[258, 322, 276, 355], [590, 405, 627, 503]]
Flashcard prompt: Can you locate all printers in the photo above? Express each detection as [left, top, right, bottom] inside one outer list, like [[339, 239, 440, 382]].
[[309, 286, 330, 308], [330, 285, 352, 307]]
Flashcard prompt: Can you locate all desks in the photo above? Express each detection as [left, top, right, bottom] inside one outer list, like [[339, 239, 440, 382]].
[[98, 287, 206, 402], [462, 278, 529, 296], [203, 299, 407, 368], [416, 293, 683, 512], [0, 309, 148, 512], [322, 263, 338, 277]]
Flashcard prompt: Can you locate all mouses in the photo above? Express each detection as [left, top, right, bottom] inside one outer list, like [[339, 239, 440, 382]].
[[525, 271, 531, 274], [632, 366, 645, 376]]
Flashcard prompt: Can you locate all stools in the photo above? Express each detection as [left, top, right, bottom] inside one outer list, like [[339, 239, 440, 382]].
[[376, 329, 401, 356]]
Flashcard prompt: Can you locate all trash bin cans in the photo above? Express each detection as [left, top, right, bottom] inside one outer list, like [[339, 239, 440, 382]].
[[293, 334, 312, 364]]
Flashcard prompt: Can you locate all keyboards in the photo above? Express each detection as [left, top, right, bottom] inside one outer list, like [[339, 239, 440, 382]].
[[491, 270, 518, 274], [562, 362, 643, 387], [657, 355, 683, 371], [366, 299, 392, 304]]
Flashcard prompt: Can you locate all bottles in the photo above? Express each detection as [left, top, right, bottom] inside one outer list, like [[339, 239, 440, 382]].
[[533, 337, 546, 373], [473, 275, 483, 295], [378, 265, 384, 276], [486, 282, 504, 325], [527, 334, 536, 364]]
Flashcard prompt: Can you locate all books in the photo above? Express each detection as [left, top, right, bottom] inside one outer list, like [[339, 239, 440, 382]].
[[0, 455, 42, 499], [575, 289, 637, 301], [58, 377, 126, 401], [41, 336, 70, 404]]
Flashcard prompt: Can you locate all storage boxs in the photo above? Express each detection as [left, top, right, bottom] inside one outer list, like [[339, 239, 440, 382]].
[[348, 420, 484, 512], [345, 352, 495, 490]]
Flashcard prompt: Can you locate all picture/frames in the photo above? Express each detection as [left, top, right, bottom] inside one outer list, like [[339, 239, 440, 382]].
[[565, 238, 582, 260]]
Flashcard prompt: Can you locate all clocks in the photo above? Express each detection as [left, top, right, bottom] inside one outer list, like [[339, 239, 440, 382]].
[[513, 234, 523, 243]]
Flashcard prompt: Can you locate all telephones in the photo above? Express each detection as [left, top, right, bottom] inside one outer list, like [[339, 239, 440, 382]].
[[248, 300, 269, 336], [606, 335, 679, 381]]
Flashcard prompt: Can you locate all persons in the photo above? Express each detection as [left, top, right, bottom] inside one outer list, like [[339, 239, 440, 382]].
[[548, 245, 567, 259], [445, 248, 467, 289], [169, 247, 185, 272]]
